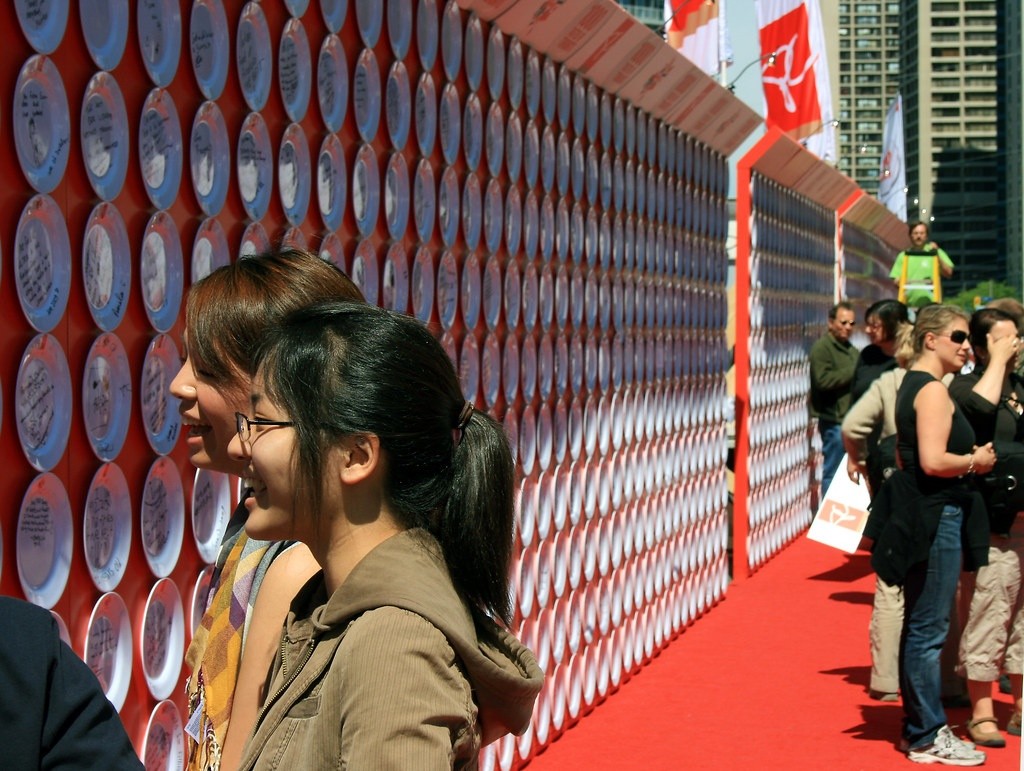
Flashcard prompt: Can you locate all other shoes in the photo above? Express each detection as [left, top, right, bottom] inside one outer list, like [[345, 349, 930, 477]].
[[965, 717, 1005, 747], [999, 680, 1012, 693], [953, 697, 971, 708], [870, 689, 898, 702], [942, 680, 964, 705], [1006, 713, 1021, 736]]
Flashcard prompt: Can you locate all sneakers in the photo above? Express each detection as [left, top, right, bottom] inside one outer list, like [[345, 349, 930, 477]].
[[908, 723, 986, 766], [899, 726, 976, 751]]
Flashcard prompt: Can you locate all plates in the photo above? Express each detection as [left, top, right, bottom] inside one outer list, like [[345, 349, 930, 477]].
[[0, 0, 726, 771], [748, 167, 899, 570]]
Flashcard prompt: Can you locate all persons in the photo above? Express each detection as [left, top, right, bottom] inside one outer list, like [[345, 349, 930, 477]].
[[0, 594, 144, 771], [225, 299, 545, 771], [811, 303, 860, 497], [168, 247, 368, 771], [888, 223, 954, 320], [841, 296, 1024, 764]]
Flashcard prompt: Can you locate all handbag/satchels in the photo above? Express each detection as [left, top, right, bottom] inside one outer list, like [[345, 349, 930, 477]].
[[987, 440, 1024, 536]]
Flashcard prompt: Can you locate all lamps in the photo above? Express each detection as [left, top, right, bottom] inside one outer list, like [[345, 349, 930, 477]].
[[722, 50, 777, 92], [799, 117, 935, 228]]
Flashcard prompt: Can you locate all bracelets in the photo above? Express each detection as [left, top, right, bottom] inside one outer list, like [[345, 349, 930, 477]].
[[966, 455, 975, 473]]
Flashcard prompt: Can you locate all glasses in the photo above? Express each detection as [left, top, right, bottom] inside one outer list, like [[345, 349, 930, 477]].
[[865, 321, 884, 333], [931, 330, 971, 344], [832, 318, 856, 326], [233, 410, 300, 441]]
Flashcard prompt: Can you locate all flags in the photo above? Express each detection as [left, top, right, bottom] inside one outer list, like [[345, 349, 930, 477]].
[[665, 0, 734, 76], [878, 96, 907, 225], [755, 0, 836, 161]]
